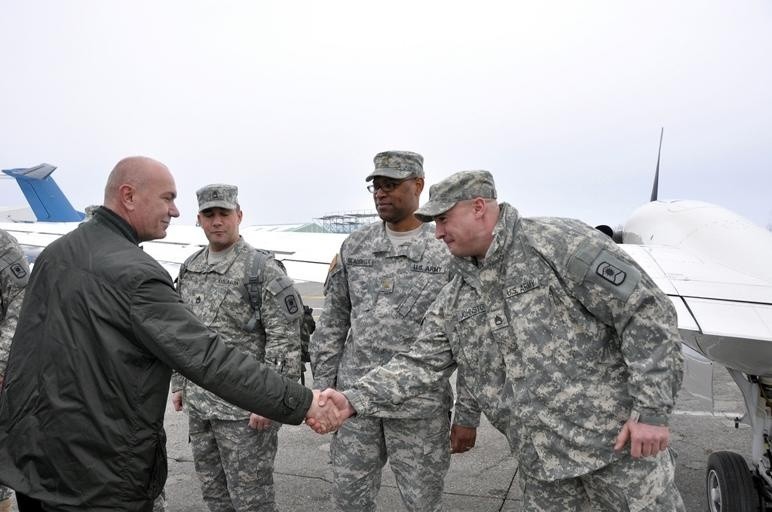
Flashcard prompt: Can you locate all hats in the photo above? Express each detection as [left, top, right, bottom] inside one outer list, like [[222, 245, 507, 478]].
[[196, 185, 239, 213], [413, 170, 496, 222], [366, 151, 424, 179]]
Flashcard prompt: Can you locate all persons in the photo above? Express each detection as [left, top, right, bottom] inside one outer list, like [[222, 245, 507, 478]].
[[171, 184, 305, 511], [306, 169, 687, 512], [310, 150, 481, 512], [1, 227, 30, 511], [0, 155, 344, 512]]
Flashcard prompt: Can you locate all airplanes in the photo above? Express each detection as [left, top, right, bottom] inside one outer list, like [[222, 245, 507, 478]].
[[1, 124, 772, 512]]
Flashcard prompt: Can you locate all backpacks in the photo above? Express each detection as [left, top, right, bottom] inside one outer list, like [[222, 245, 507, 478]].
[[247, 248, 315, 371]]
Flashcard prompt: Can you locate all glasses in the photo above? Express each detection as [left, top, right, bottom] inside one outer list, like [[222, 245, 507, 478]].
[[367, 180, 406, 192]]
